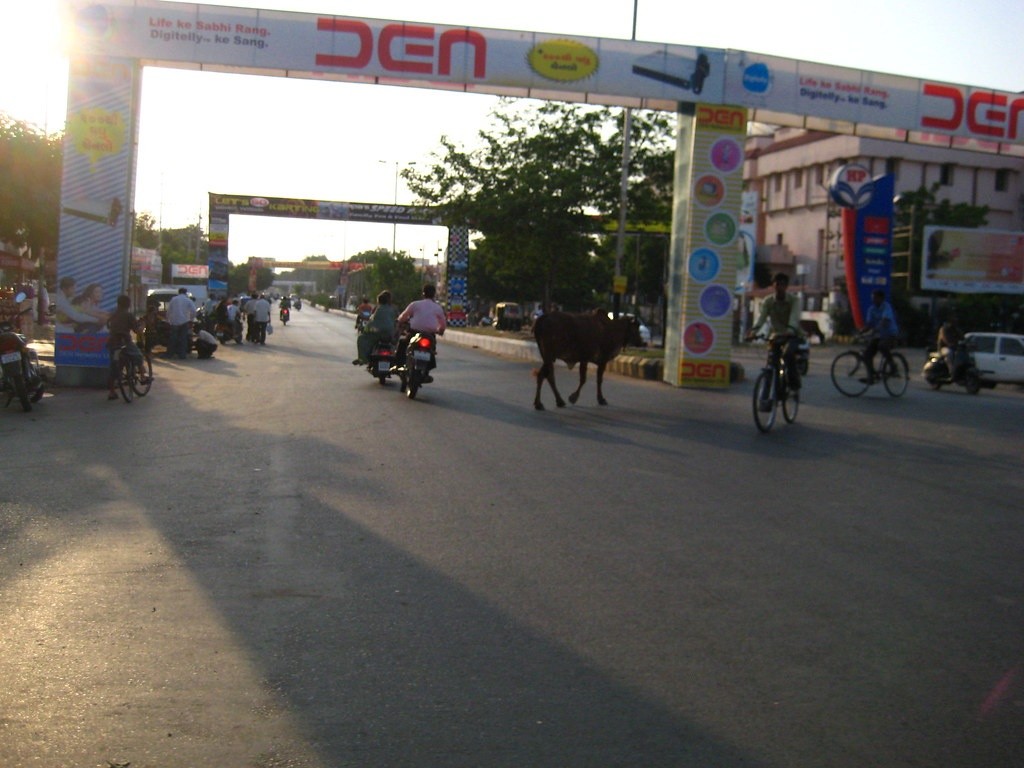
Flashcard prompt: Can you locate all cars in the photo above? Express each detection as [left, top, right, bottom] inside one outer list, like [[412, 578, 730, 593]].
[[608, 312, 651, 344]]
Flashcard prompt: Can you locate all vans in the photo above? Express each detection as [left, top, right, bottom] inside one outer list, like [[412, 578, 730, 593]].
[[959, 331, 1024, 389]]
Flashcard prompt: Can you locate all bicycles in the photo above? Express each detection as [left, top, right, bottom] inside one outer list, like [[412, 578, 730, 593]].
[[113, 317, 153, 402], [830, 336, 911, 399], [743, 332, 801, 434]]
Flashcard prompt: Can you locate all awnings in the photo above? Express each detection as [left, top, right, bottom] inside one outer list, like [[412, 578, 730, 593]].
[[0, 250, 36, 273]]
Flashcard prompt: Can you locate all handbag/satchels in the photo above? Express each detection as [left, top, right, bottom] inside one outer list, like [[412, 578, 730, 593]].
[[267, 321, 273, 335]]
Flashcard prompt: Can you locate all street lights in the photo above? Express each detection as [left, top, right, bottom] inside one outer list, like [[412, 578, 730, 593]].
[[378, 159, 416, 258]]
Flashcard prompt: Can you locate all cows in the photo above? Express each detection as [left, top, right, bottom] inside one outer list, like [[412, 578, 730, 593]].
[[532, 308, 648, 411]]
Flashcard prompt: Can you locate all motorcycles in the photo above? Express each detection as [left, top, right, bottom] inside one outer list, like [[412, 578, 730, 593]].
[[192, 306, 214, 332], [920, 333, 983, 395], [294, 303, 301, 311], [149, 289, 196, 333], [356, 311, 372, 332], [790, 338, 813, 375], [0, 291, 47, 413], [369, 336, 397, 383], [216, 318, 245, 344], [280, 307, 289, 326], [494, 301, 525, 332], [388, 318, 443, 397]]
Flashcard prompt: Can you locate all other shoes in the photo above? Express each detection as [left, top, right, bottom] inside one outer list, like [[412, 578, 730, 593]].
[[141, 377, 154, 385], [108, 394, 118, 400], [859, 378, 875, 385]]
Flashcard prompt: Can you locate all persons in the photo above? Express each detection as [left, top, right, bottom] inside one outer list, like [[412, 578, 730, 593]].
[[107, 295, 155, 400], [352, 290, 400, 373], [204, 291, 301, 346], [163, 288, 195, 359], [55, 275, 112, 334], [530, 305, 543, 335], [745, 273, 808, 412], [937, 316, 965, 380], [853, 290, 900, 384], [388, 284, 446, 383], [192, 325, 217, 359]]
